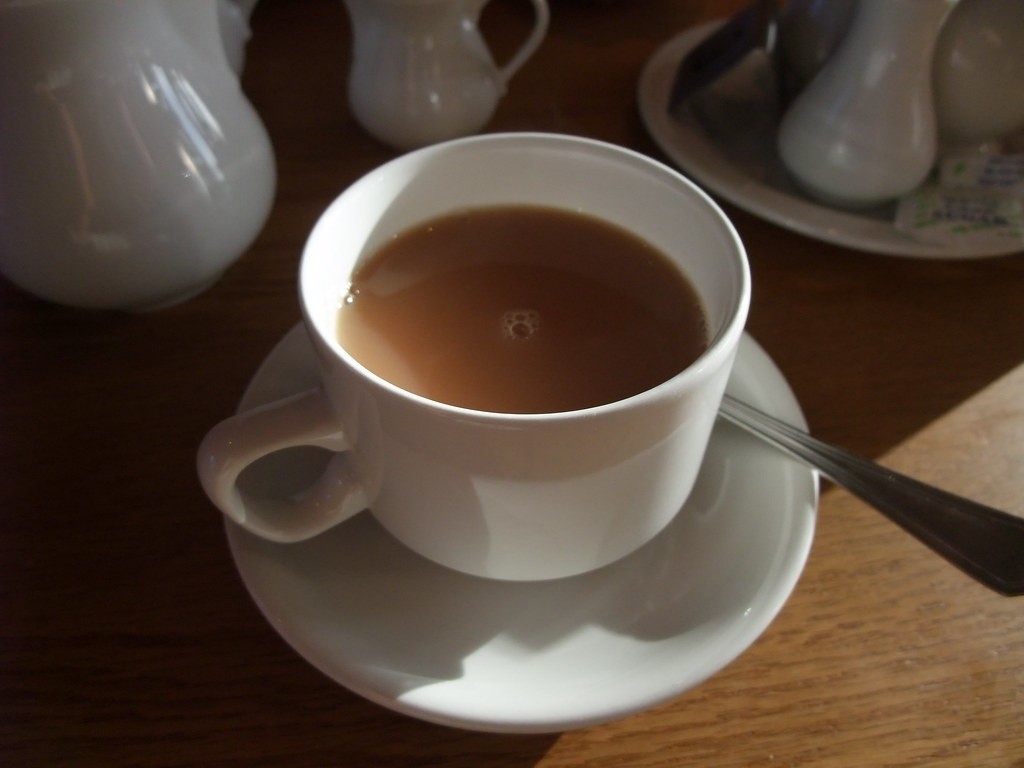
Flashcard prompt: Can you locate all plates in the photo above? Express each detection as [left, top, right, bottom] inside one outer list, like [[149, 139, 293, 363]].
[[640, 20, 1024, 261], [224, 321, 819, 735]]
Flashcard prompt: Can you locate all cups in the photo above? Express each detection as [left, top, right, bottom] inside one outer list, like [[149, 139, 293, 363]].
[[0, 0, 275, 318], [199, 133, 753, 579], [345, 0, 551, 155]]
